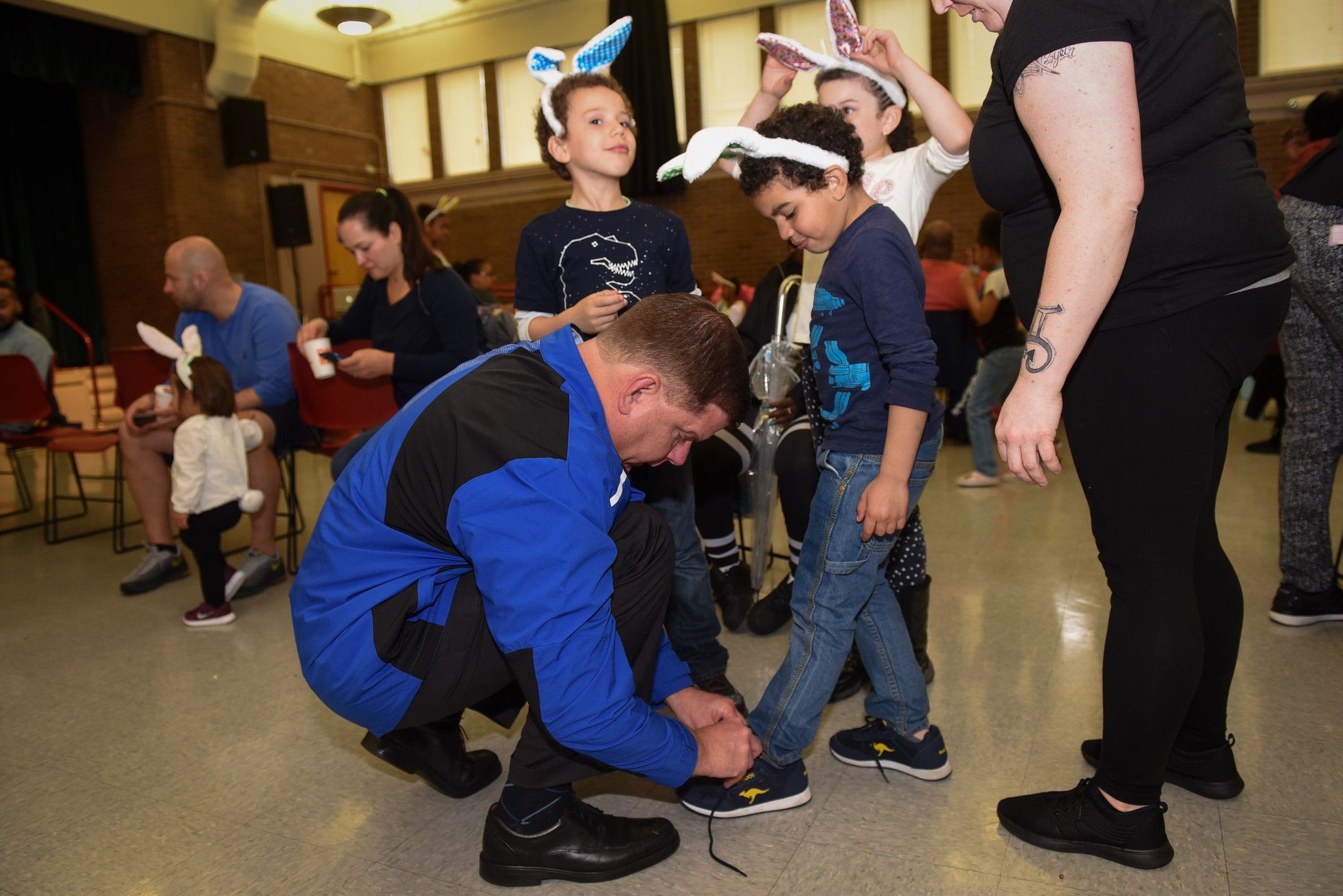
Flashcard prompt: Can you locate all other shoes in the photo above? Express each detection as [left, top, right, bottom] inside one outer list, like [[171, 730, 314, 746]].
[[1245, 431, 1282, 454], [707, 560, 754, 629], [1267, 577, 1343, 626], [747, 572, 793, 635], [700, 678, 749, 718], [956, 470, 999, 488]]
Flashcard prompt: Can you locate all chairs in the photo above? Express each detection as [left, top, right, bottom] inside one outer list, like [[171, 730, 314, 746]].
[[0, 353, 85, 540], [286, 337, 400, 581], [44, 338, 310, 563]]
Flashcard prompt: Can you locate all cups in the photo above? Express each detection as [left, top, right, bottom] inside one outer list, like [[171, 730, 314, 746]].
[[154, 384, 172, 428], [301, 337, 336, 380]]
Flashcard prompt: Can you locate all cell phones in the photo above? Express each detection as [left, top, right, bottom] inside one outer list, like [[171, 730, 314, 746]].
[[320, 352, 346, 364]]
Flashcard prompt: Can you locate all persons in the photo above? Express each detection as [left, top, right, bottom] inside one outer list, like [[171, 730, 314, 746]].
[[120, 236, 305, 600], [934, 0, 1293, 870], [512, 16, 751, 719], [290, 292, 763, 887], [711, 270, 747, 328], [297, 185, 489, 482], [137, 321, 263, 626], [656, 101, 953, 819], [1241, 86, 1343, 627], [0, 257, 56, 435], [756, 0, 973, 706], [919, 210, 1027, 487], [418, 194, 515, 349]]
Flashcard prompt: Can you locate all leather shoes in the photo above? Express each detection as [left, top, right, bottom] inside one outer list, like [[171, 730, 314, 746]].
[[362, 712, 502, 798], [480, 791, 680, 887]]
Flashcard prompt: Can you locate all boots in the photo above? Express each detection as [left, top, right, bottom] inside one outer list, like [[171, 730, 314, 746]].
[[870, 573, 935, 693], [828, 636, 868, 704]]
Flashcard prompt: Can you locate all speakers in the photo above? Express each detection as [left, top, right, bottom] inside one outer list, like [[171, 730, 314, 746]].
[[265, 183, 313, 248], [217, 94, 270, 167]]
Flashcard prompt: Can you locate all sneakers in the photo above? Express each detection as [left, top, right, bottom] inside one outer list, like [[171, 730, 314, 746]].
[[1081, 733, 1245, 800], [998, 778, 1174, 869], [119, 539, 190, 595], [232, 545, 287, 600], [223, 565, 246, 601], [182, 601, 236, 627], [828, 715, 952, 785], [679, 758, 811, 878]]
